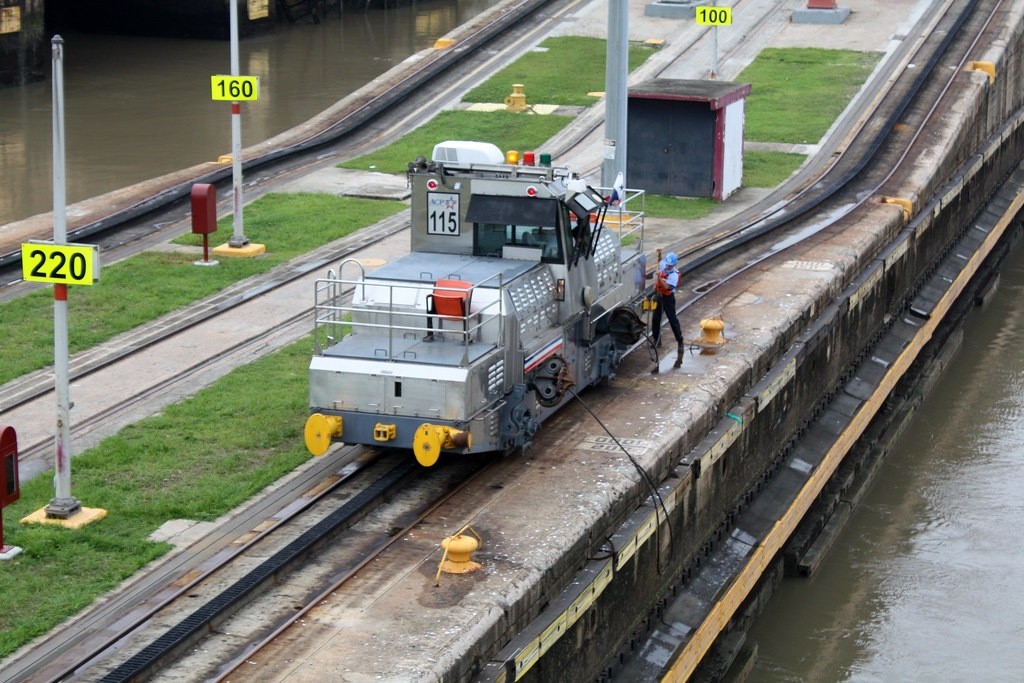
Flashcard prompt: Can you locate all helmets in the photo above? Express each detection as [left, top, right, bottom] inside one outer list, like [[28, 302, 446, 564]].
[[664, 252, 678, 266]]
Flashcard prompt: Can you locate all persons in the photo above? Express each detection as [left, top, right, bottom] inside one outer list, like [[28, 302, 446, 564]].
[[650, 249, 685, 354]]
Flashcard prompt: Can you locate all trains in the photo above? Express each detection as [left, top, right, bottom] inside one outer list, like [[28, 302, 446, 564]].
[[305, 140, 655, 468]]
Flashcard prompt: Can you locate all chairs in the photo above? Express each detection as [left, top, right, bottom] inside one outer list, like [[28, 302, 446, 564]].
[[423, 279, 474, 345]]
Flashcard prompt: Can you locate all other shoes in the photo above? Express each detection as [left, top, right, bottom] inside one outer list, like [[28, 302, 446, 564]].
[[650, 340, 662, 348], [678, 342, 683, 353]]
